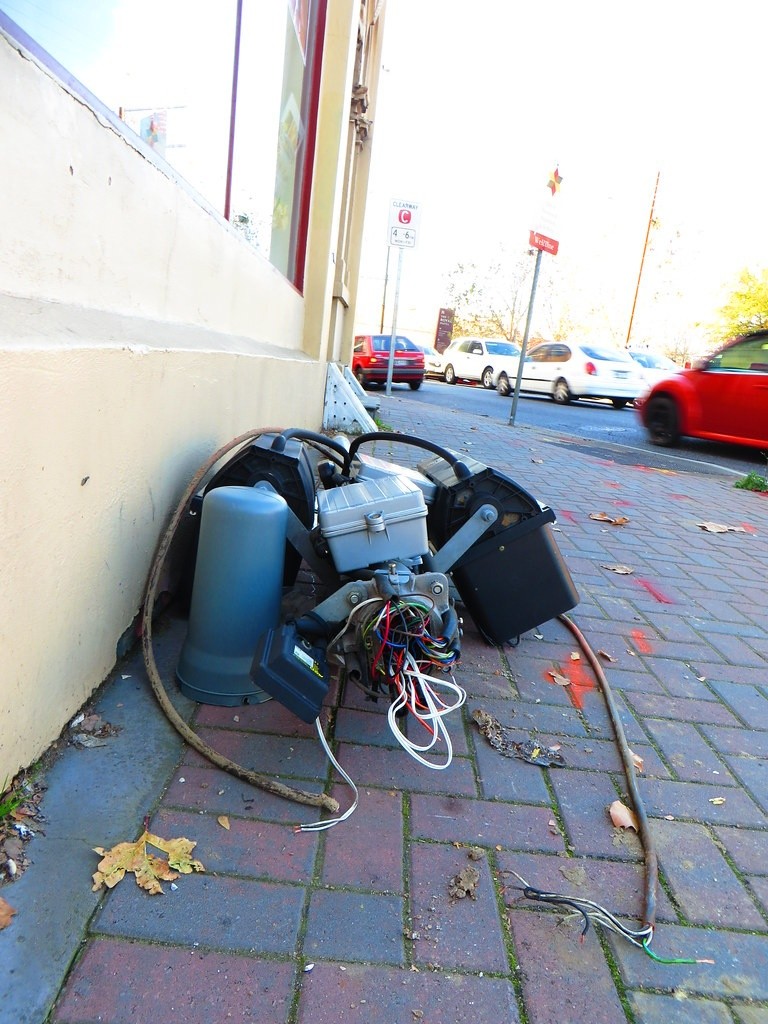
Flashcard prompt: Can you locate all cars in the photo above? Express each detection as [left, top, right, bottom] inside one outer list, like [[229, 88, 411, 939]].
[[623, 348, 684, 384], [491, 339, 649, 409], [441, 336, 531, 390], [418, 343, 446, 382], [635, 329, 768, 449], [352, 334, 425, 391]]
